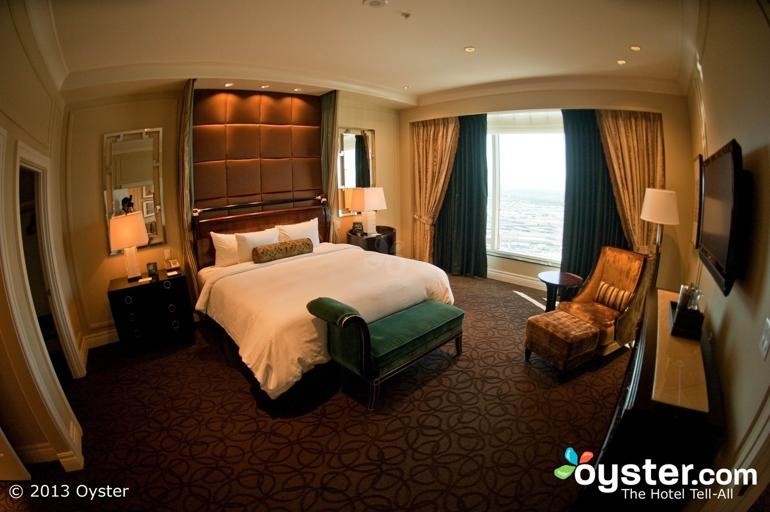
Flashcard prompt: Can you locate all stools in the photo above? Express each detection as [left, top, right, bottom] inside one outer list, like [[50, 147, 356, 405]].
[[524, 310, 599, 379]]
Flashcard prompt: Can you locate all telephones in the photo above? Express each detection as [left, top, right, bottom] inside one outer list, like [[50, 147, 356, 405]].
[[164, 258, 180, 271]]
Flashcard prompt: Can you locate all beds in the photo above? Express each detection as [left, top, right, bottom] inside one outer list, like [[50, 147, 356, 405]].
[[193, 196, 455, 419]]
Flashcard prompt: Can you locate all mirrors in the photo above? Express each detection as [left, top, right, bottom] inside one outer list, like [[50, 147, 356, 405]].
[[337, 127, 377, 217], [104, 127, 166, 255]]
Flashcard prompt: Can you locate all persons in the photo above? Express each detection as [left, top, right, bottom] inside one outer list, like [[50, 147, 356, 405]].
[[117, 197, 137, 216]]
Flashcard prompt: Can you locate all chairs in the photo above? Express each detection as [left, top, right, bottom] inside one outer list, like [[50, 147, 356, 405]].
[[556, 246, 645, 369], [306, 297, 463, 412]]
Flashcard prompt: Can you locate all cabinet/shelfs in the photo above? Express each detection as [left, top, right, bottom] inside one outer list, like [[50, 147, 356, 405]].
[[577, 289, 726, 512]]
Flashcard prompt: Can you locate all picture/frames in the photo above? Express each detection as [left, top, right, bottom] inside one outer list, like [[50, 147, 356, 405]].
[[143, 201, 154, 217], [142, 186, 153, 197]]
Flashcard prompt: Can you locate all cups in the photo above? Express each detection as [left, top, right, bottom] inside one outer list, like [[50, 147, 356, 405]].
[[674, 284, 701, 324]]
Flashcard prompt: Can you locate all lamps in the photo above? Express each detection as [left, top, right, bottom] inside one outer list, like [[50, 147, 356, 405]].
[[338, 189, 349, 213], [345, 187, 386, 233], [641, 188, 680, 287], [109, 211, 149, 283]]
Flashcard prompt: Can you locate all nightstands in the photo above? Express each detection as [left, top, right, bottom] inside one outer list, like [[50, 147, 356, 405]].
[[348, 226, 397, 256], [108, 270, 195, 359]]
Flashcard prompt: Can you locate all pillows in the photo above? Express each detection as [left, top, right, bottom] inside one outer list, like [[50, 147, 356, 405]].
[[595, 282, 632, 311], [235, 229, 279, 263], [253, 239, 312, 263], [275, 218, 319, 248], [211, 232, 239, 266]]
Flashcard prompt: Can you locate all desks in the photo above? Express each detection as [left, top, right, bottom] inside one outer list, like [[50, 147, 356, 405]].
[[538, 271, 583, 312]]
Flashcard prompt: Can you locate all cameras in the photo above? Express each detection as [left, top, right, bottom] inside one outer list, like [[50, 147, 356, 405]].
[[127, 195, 133, 207]]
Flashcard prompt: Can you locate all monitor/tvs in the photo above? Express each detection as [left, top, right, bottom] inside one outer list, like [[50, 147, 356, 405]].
[[700, 138, 751, 295]]
